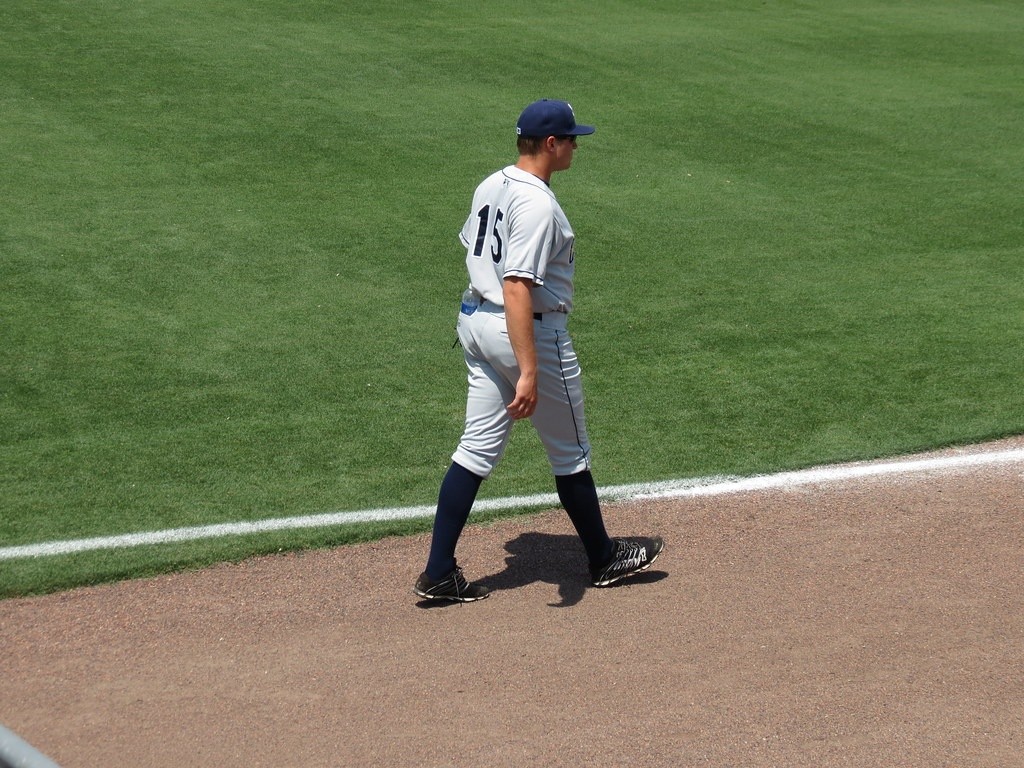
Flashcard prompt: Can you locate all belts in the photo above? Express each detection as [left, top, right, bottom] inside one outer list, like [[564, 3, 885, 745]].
[[479, 296, 542, 321]]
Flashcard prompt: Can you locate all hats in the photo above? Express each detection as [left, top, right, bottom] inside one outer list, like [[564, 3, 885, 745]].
[[516, 99, 595, 135]]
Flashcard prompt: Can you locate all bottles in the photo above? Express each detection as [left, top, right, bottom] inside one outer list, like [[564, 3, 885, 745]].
[[461, 283, 481, 315]]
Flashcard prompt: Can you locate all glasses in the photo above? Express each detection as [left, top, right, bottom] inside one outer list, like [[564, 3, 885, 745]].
[[554, 134, 577, 143]]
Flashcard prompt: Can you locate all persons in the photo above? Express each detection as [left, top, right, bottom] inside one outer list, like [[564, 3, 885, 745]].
[[414, 100, 665, 604]]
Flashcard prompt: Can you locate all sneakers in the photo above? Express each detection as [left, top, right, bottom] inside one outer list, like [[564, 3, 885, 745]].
[[413, 557, 489, 601], [588, 537, 664, 586]]
